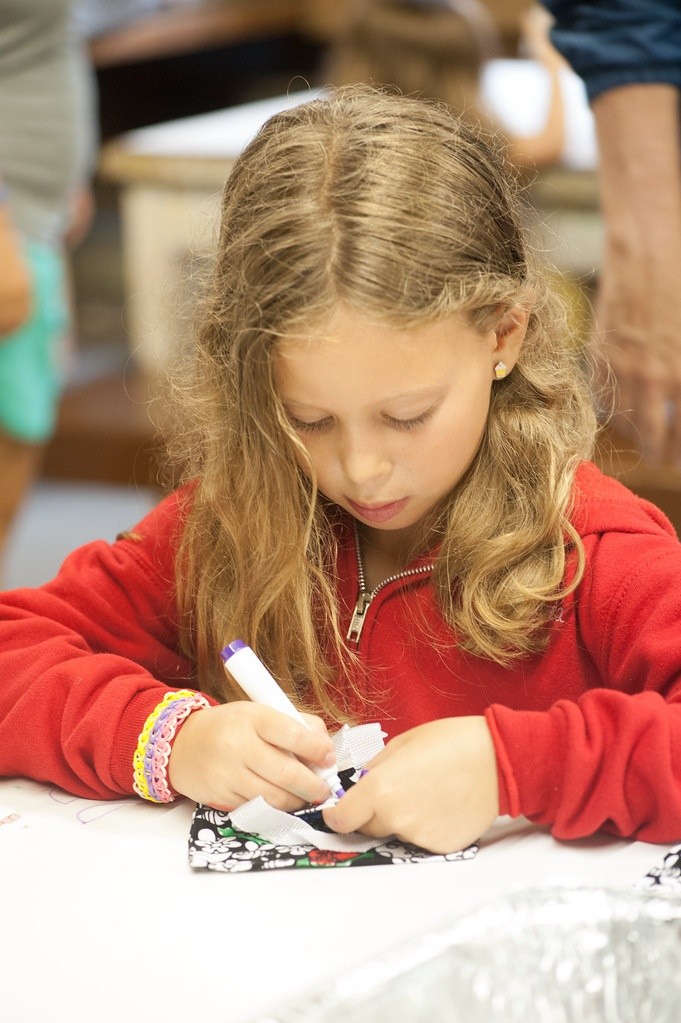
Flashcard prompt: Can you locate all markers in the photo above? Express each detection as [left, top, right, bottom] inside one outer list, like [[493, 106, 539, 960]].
[[220, 638, 349, 800]]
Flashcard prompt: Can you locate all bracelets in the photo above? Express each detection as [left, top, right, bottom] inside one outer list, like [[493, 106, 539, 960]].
[[131, 688, 211, 807]]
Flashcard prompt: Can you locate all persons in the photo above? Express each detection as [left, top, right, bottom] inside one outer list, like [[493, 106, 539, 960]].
[[0, 81, 681, 854], [320, 0, 567, 173], [1, 0, 103, 548], [542, 0, 681, 538]]
